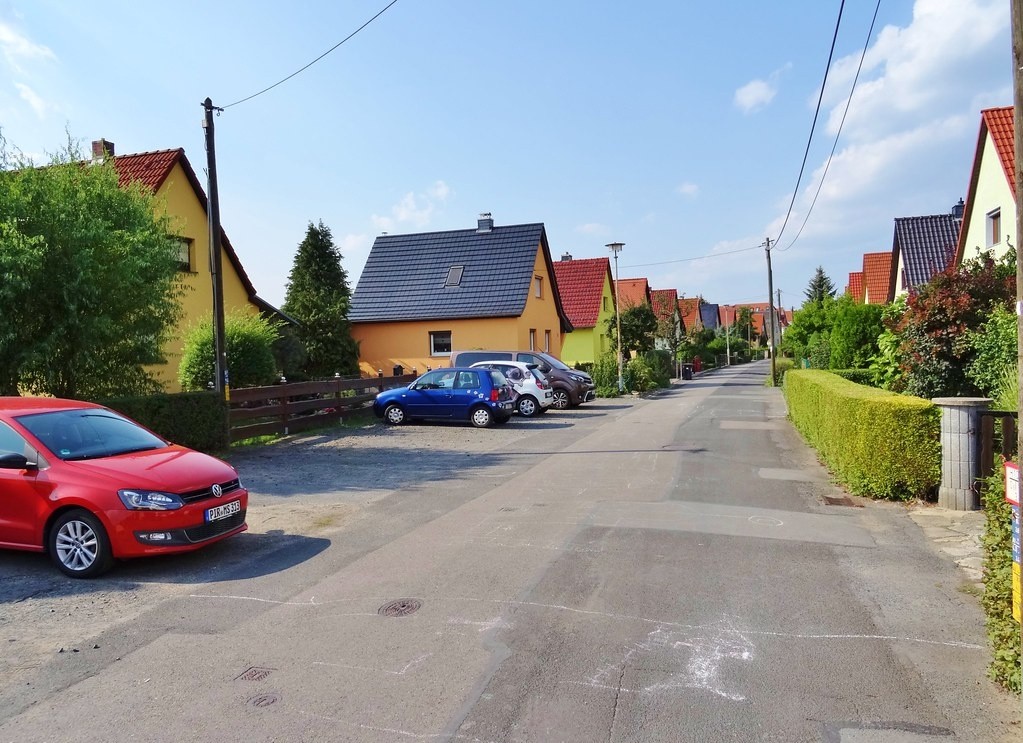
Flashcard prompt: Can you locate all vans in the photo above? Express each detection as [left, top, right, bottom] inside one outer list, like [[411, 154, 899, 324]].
[[450, 350, 596, 410]]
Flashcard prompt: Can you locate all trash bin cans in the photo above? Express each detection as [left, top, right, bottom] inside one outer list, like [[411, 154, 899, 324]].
[[682, 362, 695, 380]]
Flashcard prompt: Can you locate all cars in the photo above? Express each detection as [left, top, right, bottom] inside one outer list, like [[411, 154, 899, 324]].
[[374, 367, 518, 428], [439, 360, 554, 418], [0, 396, 249, 578]]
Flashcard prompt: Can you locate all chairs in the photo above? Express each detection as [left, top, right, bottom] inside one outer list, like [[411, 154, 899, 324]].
[[462, 374, 472, 388]]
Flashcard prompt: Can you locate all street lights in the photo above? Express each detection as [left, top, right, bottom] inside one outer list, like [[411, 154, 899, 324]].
[[724, 304, 730, 366], [605, 242, 626, 393]]
